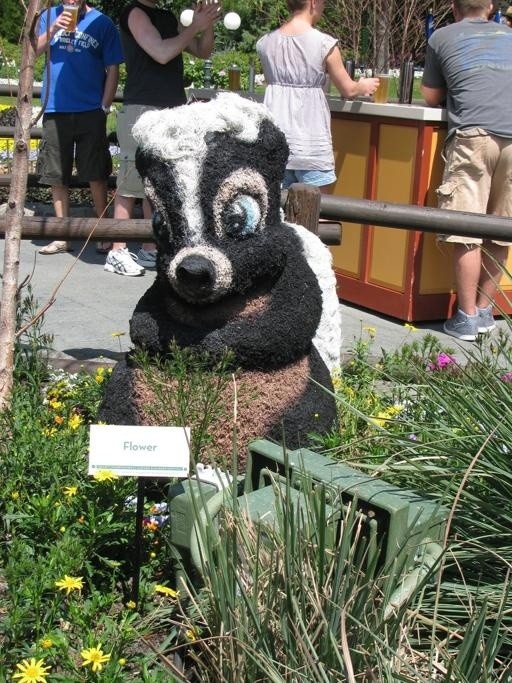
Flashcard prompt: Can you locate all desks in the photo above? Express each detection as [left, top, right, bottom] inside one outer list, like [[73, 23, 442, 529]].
[[191, 89, 512, 323]]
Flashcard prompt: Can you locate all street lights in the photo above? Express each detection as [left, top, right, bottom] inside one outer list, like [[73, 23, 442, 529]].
[[177, 7, 243, 87]]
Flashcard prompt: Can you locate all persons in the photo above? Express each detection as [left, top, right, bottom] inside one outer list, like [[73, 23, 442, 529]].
[[102, 0, 225, 276], [418, 0, 512, 342], [254, 0, 381, 188], [30, 0, 127, 257]]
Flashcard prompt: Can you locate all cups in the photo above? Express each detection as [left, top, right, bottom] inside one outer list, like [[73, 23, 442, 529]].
[[373, 74, 391, 102], [228, 63, 242, 89], [62, 3, 80, 31]]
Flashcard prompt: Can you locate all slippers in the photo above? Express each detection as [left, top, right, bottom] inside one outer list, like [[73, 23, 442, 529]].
[[39, 243, 74, 255]]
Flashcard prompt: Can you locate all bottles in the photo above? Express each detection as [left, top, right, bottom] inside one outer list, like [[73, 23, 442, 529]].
[[398, 55, 415, 104], [495, 7, 503, 25], [341, 56, 355, 101], [427, 7, 434, 39]]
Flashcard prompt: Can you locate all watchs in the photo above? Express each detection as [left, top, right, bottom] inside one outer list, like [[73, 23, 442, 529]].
[[102, 105, 111, 116]]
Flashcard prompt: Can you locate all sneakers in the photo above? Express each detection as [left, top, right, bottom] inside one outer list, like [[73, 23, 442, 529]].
[[443, 309, 479, 342], [104, 248, 145, 277], [477, 305, 496, 334], [137, 249, 158, 268]]
